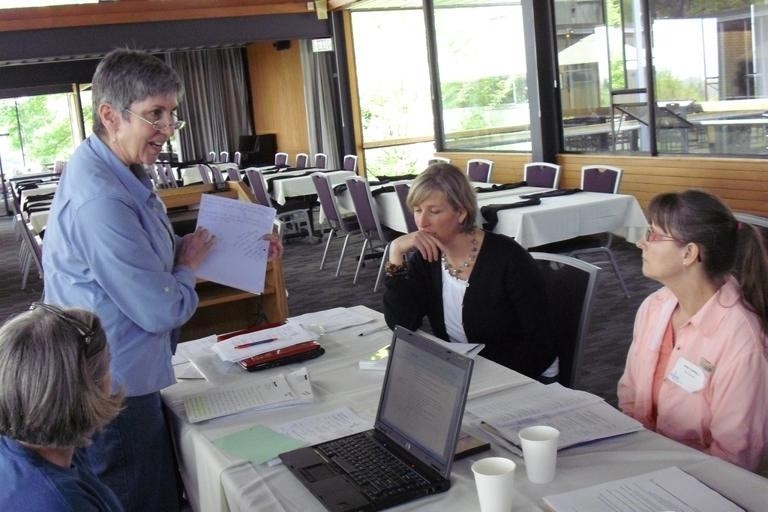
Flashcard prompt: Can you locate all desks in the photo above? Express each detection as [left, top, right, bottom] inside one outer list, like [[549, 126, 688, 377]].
[[319, 176, 649, 251], [158, 304, 768, 512]]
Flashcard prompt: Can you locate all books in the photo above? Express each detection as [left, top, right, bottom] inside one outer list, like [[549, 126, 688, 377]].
[[359, 343, 486, 370], [481, 419, 624, 451]]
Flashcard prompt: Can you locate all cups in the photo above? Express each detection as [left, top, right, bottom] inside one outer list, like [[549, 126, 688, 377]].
[[519, 425, 560, 483], [471, 458, 517, 512]]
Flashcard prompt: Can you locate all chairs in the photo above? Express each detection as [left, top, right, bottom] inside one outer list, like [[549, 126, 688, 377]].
[[427, 156, 451, 170], [524, 163, 559, 186], [467, 158, 494, 181], [731, 211, 768, 258], [344, 177, 393, 291], [560, 165, 632, 298], [395, 184, 419, 233], [528, 250, 601, 388]]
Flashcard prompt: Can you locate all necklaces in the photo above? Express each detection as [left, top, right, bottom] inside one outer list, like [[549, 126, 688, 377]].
[[440, 228, 479, 277]]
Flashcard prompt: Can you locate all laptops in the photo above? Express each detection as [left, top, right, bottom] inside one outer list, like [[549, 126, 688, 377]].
[[279, 325, 474, 511], [216, 322, 325, 372]]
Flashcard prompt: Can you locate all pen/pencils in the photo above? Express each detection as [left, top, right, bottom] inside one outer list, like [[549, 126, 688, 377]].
[[234, 338, 279, 349], [358, 325, 389, 337]]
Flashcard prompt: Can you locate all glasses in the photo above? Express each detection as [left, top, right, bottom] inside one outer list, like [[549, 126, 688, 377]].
[[126, 107, 186, 131], [29, 302, 95, 345], [645, 229, 702, 262]]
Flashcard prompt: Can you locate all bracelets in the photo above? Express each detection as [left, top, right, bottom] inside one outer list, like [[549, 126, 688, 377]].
[[384, 261, 408, 274]]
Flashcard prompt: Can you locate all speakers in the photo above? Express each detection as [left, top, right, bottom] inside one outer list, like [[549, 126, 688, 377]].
[[273, 40, 290, 51]]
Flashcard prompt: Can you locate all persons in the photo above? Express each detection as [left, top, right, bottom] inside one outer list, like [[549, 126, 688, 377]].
[[381, 162, 567, 387], [43, 47, 285, 512], [0, 302, 127, 512], [616, 190, 767, 477]]
